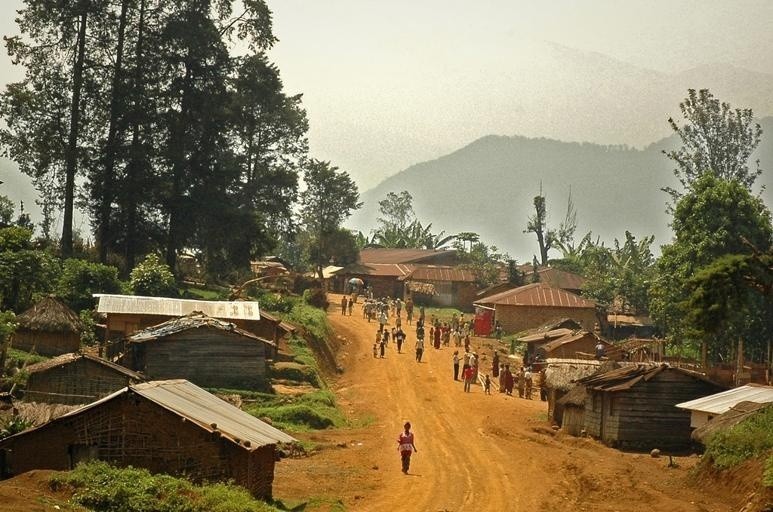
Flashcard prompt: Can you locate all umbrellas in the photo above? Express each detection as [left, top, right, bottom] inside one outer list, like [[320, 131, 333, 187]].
[[348, 278, 363, 286]]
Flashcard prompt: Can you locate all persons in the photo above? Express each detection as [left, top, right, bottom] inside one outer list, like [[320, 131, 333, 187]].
[[341, 282, 470, 363], [452, 348, 546, 400], [397, 421, 417, 474]]
